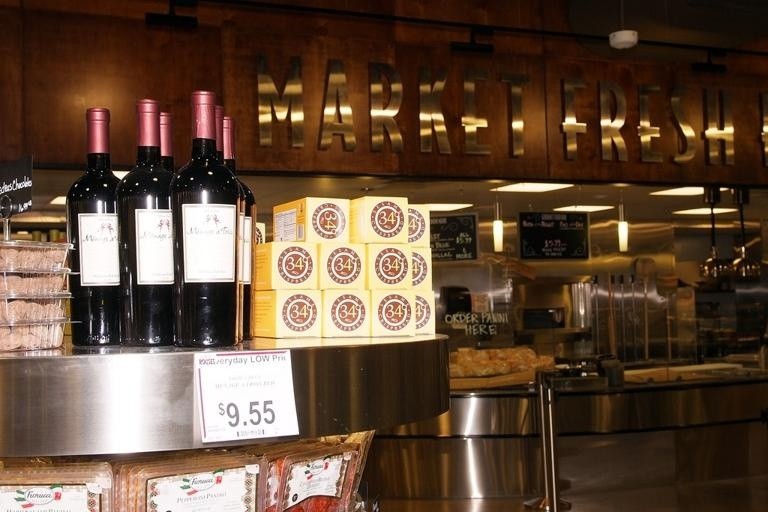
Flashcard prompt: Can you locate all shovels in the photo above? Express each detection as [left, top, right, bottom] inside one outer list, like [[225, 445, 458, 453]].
[[636, 258, 656, 361]]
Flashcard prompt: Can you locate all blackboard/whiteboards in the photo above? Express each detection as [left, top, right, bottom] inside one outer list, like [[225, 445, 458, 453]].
[[430, 212, 480, 266], [515, 209, 591, 263]]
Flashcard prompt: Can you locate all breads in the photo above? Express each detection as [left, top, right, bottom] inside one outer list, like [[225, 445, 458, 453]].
[[449, 346, 555, 377]]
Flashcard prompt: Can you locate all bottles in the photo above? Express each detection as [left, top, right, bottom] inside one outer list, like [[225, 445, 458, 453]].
[[67, 106, 120, 345], [119, 89, 261, 345]]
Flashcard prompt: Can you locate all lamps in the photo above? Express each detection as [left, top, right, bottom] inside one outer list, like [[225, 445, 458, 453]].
[[699, 187, 734, 280], [730, 188, 762, 282], [617, 204, 629, 252], [492, 201, 504, 253]]
